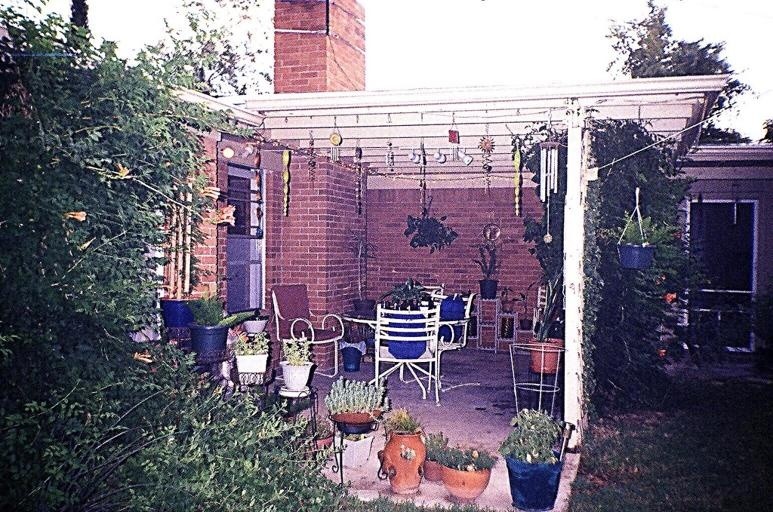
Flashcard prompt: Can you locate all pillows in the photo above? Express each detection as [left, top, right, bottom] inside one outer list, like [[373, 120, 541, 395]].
[[389, 310, 427, 359], [440, 295, 464, 342]]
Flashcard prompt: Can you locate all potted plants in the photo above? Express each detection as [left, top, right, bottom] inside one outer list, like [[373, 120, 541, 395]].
[[525, 261, 563, 375], [345, 225, 377, 316], [516, 281, 532, 329], [187, 291, 315, 398], [599, 208, 674, 269], [500, 284, 523, 313], [475, 242, 503, 294], [297, 378, 496, 507], [500, 405, 567, 509], [381, 275, 432, 308]]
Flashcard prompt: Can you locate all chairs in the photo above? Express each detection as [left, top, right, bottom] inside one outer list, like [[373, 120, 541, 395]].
[[373, 303, 440, 406], [438, 293, 479, 392], [270, 282, 346, 379]]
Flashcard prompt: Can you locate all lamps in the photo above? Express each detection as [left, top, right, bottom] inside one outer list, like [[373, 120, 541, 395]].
[[403, 146, 474, 168], [240, 143, 254, 159]]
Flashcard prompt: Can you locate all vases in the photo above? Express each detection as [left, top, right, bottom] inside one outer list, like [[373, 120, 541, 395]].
[[156, 298, 191, 332]]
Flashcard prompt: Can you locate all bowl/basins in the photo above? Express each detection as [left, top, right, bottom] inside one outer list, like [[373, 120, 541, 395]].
[[243, 320, 268, 333]]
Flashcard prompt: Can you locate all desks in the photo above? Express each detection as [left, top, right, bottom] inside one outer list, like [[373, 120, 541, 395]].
[[343, 316, 468, 383]]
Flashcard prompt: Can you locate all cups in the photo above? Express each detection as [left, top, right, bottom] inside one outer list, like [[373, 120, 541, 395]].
[[381, 300, 413, 317]]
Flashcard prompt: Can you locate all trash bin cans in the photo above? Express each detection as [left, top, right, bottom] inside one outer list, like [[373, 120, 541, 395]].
[[339, 341, 365, 372]]
